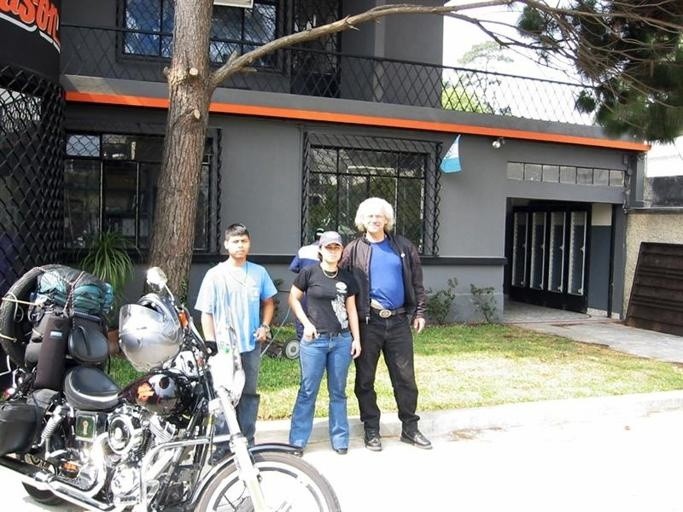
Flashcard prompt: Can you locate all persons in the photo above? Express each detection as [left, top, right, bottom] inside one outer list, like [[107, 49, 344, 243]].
[[289, 227, 327, 356], [337, 197, 432, 451], [288, 231, 362, 460], [193, 224, 278, 465]]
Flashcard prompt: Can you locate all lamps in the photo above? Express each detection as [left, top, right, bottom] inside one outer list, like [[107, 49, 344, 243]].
[[491, 137, 505, 149]]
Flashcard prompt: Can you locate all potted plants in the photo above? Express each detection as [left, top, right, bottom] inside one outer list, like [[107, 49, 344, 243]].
[[77, 228, 144, 354]]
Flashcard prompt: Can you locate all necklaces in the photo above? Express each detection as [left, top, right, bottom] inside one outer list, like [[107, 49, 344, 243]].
[[321, 262, 339, 278]]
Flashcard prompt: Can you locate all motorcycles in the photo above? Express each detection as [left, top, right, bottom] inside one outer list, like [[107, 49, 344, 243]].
[[0, 266, 342, 512]]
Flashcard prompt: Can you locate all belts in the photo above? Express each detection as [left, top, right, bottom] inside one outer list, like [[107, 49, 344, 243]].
[[371, 307, 408, 319]]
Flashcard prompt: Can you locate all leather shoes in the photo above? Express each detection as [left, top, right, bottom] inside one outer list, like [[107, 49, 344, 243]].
[[212, 440, 230, 464], [335, 447, 347, 455], [362, 429, 384, 451], [399, 429, 432, 449], [290, 446, 306, 457], [240, 440, 263, 459]]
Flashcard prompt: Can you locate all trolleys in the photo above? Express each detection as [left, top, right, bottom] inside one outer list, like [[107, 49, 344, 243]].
[[254, 287, 301, 360]]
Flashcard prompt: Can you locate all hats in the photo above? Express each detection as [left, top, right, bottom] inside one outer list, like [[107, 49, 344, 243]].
[[319, 232, 343, 248]]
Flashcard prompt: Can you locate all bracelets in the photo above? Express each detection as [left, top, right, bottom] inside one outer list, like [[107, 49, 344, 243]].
[[262, 325, 270, 332]]
